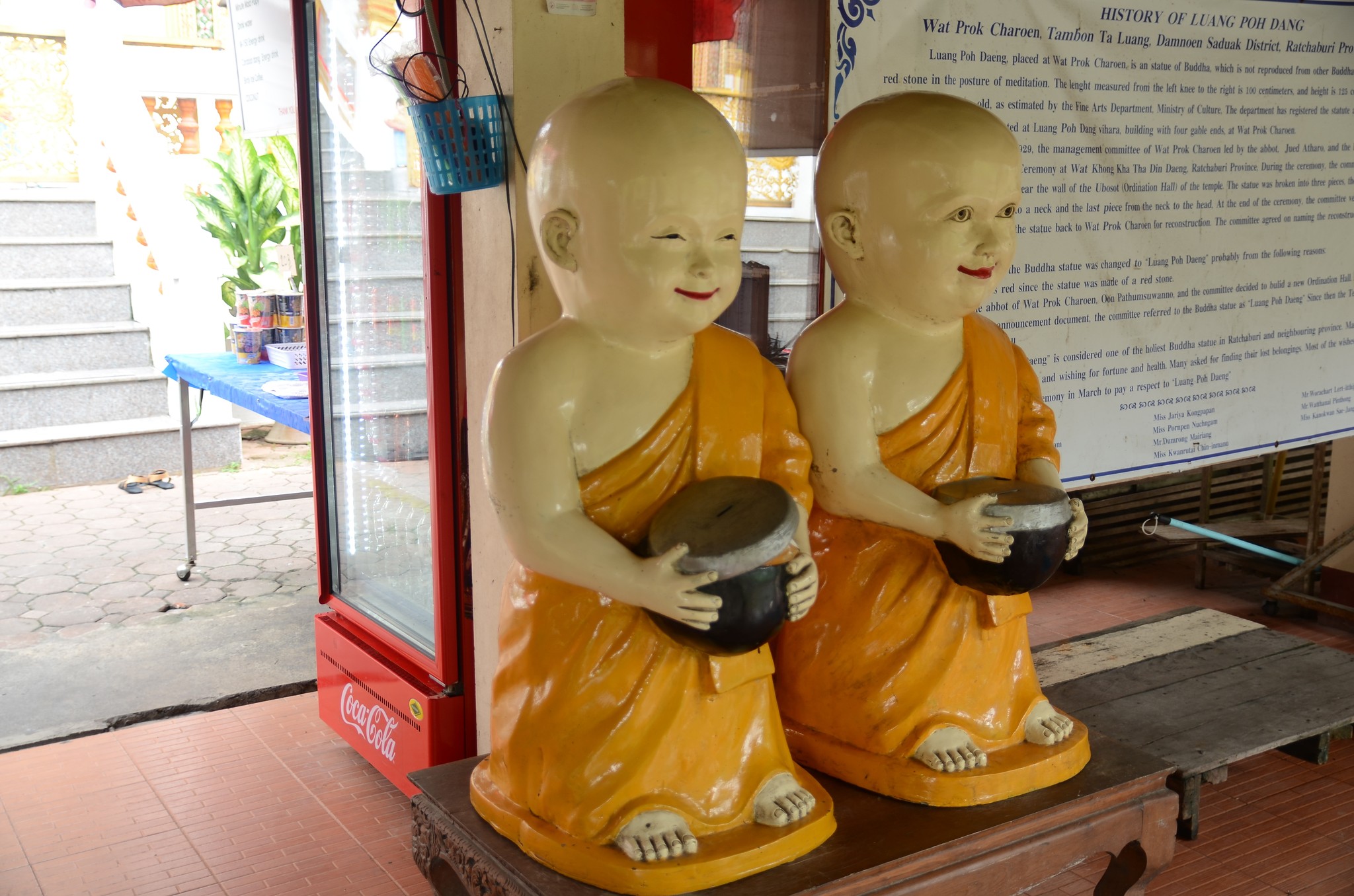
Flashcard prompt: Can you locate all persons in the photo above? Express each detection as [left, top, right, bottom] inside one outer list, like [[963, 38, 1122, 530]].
[[478, 76, 818, 862], [783, 89, 1091, 777]]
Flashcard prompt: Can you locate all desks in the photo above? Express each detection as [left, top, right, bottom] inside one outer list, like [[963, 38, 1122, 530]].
[[162, 353, 312, 578], [398, 756, 1174, 895]]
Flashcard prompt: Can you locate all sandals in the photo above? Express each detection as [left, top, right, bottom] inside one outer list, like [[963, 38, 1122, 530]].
[[118, 469, 175, 494]]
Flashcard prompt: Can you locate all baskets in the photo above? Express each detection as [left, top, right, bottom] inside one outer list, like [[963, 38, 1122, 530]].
[[265, 341, 307, 370], [404, 93, 507, 197]]
